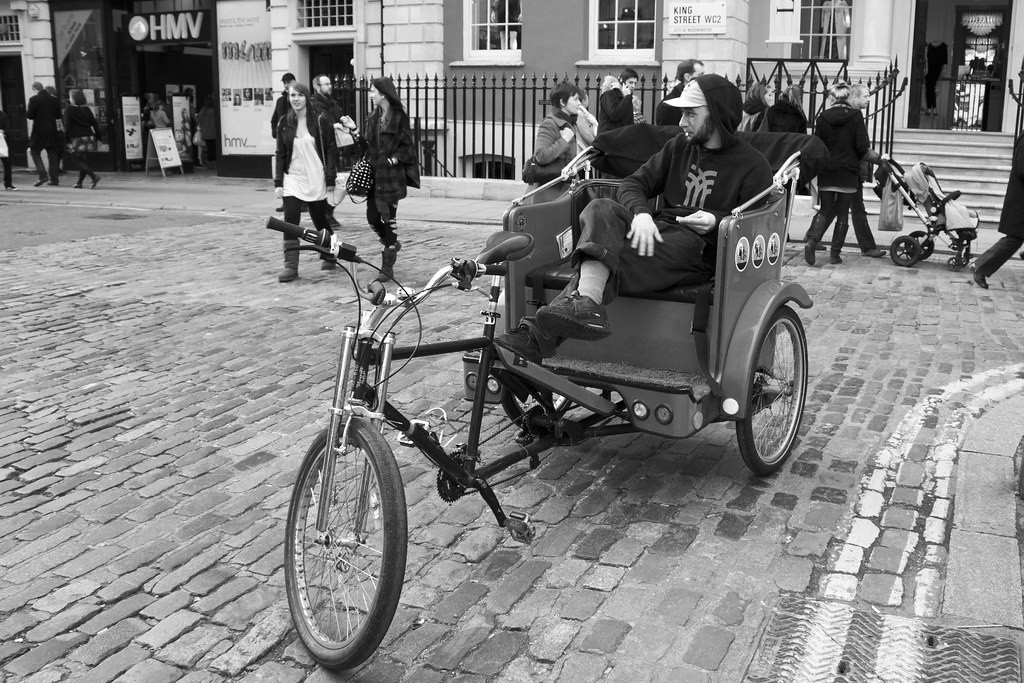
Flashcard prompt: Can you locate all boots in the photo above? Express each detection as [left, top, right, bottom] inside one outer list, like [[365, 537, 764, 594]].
[[279, 239, 300, 282], [830, 223, 848, 264], [378, 249, 397, 281], [322, 259, 335, 270], [805, 214, 828, 265], [380, 234, 401, 251]]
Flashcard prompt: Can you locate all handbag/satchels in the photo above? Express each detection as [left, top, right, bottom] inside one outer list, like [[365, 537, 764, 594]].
[[522, 116, 571, 184], [333, 122, 354, 148], [0, 132, 9, 159], [347, 146, 374, 197], [193, 131, 205, 146], [326, 179, 347, 207], [878, 175, 903, 232]]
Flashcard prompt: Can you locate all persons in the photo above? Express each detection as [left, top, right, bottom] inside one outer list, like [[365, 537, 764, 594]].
[[271, 72, 342, 282], [738, 77, 772, 133], [140, 93, 170, 128], [656, 59, 706, 127], [599, 68, 638, 135], [198, 95, 218, 168], [491, 0, 522, 50], [600, 73, 646, 124], [495, 74, 773, 365], [524, 81, 598, 204], [967, 128, 1024, 289], [803, 83, 890, 265], [766, 84, 807, 135], [233, 88, 252, 107], [181, 109, 191, 150], [0, 82, 100, 190], [340, 77, 421, 280]]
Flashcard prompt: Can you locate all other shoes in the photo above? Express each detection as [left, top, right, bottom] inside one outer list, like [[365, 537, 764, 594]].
[[813, 241, 826, 250], [300, 203, 306, 213], [5, 175, 101, 190], [276, 204, 284, 211], [865, 249, 885, 257], [969, 263, 988, 288]]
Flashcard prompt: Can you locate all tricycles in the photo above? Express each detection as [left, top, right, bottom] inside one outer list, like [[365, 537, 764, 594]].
[[262, 131, 830, 673]]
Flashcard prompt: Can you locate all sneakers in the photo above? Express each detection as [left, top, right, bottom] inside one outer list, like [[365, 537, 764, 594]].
[[536, 290, 611, 340], [495, 323, 543, 365]]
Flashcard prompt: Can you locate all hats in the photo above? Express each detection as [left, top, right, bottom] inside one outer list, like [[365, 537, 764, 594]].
[[281, 73, 295, 80], [662, 79, 708, 109]]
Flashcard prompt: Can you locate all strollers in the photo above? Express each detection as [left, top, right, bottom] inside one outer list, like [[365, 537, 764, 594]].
[[871, 157, 981, 272]]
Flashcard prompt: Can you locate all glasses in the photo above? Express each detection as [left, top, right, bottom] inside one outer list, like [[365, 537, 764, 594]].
[[319, 83, 332, 86]]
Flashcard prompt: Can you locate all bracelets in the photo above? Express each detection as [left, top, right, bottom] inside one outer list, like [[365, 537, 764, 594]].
[[387, 158, 395, 166], [352, 129, 360, 135]]
